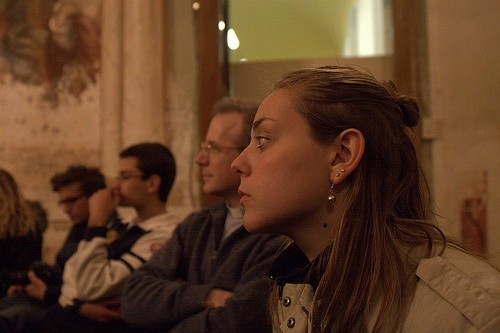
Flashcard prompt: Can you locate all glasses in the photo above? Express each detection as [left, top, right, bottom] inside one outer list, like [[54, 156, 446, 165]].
[[57, 188, 91, 208], [200, 142, 245, 153], [117, 171, 146, 181]]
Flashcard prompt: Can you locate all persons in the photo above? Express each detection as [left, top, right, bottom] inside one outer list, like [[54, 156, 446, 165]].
[[6, 166, 122, 302], [0, 170, 50, 305], [231, 66, 500, 333], [120, 98, 294, 333], [0, 143, 183, 333]]
